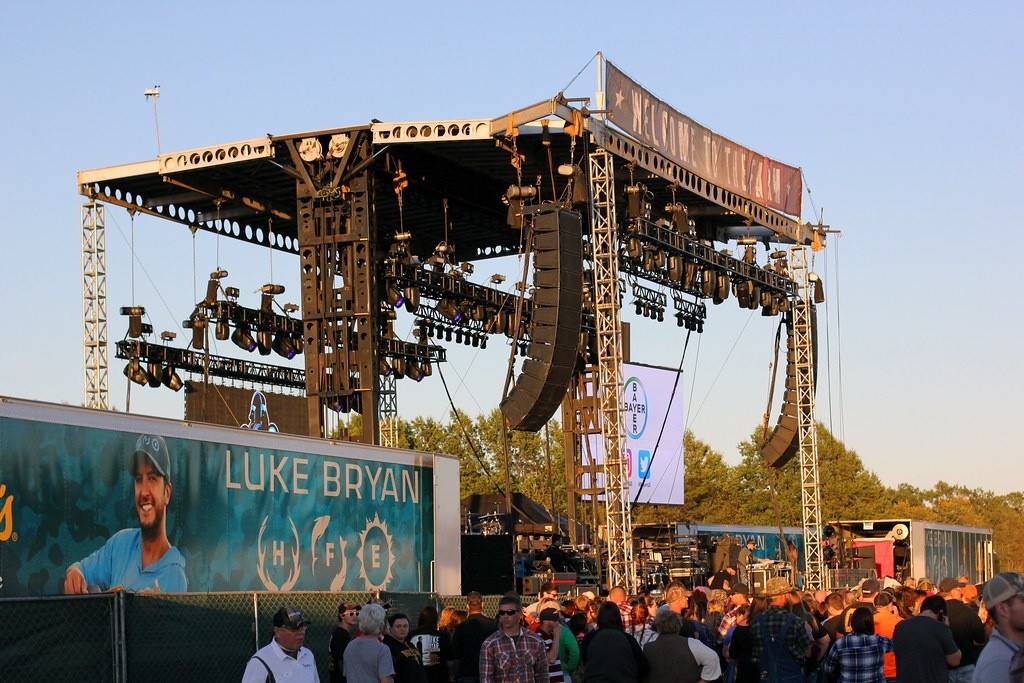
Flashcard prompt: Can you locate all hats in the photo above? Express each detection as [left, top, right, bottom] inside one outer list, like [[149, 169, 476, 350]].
[[982, 571, 1024, 610], [541, 607, 561, 622], [338, 601, 362, 614], [273, 606, 311, 630], [727, 583, 749, 596], [366, 599, 391, 608], [813, 572, 979, 627], [581, 591, 595, 601], [467, 591, 482, 605], [540, 601, 566, 612], [664, 580, 693, 603], [747, 539, 758, 545], [696, 585, 712, 602], [128, 433, 171, 482], [757, 577, 800, 598]]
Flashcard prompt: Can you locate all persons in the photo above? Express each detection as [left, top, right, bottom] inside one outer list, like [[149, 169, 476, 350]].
[[241, 605, 321, 683], [329, 572, 1024, 683], [737, 538, 770, 587], [63, 433, 189, 596], [544, 533, 567, 573]]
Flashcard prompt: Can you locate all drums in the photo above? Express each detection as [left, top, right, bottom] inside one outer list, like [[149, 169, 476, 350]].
[[648, 572, 668, 595]]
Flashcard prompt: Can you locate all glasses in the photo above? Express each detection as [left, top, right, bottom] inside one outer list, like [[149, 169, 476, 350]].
[[547, 591, 560, 599], [497, 609, 519, 616], [280, 624, 307, 632], [954, 587, 962, 592], [343, 612, 360, 617]]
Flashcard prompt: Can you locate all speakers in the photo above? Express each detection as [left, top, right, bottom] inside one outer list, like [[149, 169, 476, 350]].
[[760, 306, 817, 468], [499, 209, 583, 434], [711, 545, 741, 575], [522, 576, 540, 595], [698, 535, 712, 549]]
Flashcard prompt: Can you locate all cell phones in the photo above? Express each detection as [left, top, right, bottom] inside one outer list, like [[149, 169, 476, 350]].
[[938, 612, 944, 622]]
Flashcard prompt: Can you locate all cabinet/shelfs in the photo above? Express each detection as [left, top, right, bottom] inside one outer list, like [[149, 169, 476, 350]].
[[650, 534, 710, 587]]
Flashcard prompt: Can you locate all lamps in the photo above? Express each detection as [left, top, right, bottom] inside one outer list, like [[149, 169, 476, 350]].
[[119, 173, 825, 416]]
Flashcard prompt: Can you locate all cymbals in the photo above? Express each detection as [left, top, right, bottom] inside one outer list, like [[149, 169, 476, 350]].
[[492, 513, 506, 516], [466, 512, 480, 515], [474, 523, 493, 528], [479, 513, 494, 519]]
